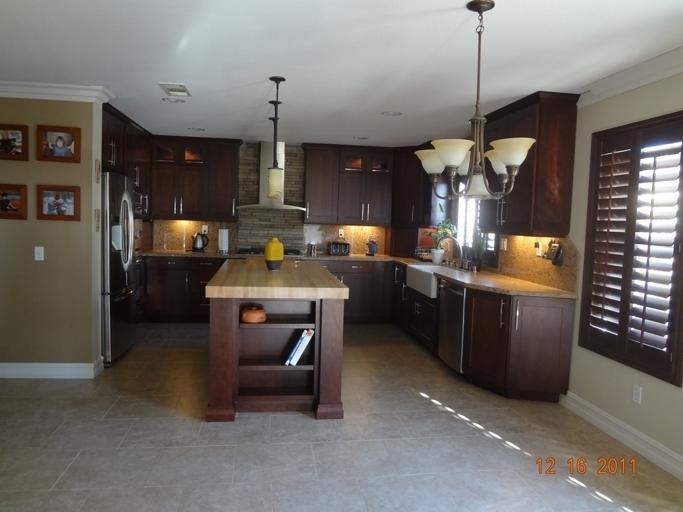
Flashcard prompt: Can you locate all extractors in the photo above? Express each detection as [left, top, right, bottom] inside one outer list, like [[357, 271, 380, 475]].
[[236, 142, 306, 211]]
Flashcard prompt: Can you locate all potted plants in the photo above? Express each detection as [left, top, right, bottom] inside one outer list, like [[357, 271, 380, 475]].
[[429, 218, 456, 265]]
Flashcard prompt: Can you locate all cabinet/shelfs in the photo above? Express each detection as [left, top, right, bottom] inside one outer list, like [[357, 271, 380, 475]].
[[102, 103, 124, 172], [301, 142, 393, 226], [393, 262, 440, 355], [479, 91, 580, 236], [203, 138, 242, 221], [466, 286, 577, 404], [141, 254, 227, 323], [232, 298, 322, 413], [152, 163, 207, 220], [124, 113, 152, 219], [393, 141, 448, 227], [316, 259, 393, 323]]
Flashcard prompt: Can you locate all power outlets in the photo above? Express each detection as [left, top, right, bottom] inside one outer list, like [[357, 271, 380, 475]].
[[339, 228, 344, 237], [202, 224, 208, 235]]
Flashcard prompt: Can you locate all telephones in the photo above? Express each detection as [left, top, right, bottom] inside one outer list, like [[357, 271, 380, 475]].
[[542, 240, 560, 260]]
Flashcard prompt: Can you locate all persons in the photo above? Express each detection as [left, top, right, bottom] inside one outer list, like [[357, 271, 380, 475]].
[[0, 138, 16, 154], [48, 136, 73, 156], [0, 192, 15, 210], [53, 195, 67, 215]]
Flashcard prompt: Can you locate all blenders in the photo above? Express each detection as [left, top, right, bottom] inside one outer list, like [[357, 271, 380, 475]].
[[216, 229, 229, 255]]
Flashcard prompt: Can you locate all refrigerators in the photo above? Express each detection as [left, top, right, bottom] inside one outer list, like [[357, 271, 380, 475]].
[[101, 171, 134, 368]]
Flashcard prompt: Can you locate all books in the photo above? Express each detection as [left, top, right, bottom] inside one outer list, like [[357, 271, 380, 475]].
[[285, 329, 315, 367]]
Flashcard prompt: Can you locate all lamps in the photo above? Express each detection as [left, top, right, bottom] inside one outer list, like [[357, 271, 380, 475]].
[[415, 0, 540, 200], [267, 76, 287, 199]]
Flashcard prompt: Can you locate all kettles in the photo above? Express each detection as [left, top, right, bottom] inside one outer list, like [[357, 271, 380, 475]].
[[191, 233, 208, 252]]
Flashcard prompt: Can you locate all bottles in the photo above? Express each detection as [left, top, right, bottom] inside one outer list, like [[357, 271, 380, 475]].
[[365, 235, 376, 256], [415, 247, 432, 262], [328, 240, 350, 256], [447, 260, 477, 272], [308, 240, 316, 256], [241, 305, 266, 323], [265, 237, 284, 271]]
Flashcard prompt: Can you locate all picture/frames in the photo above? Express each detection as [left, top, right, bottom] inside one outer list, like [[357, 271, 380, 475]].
[[0, 184, 27, 220], [36, 184, 81, 221], [37, 124, 81, 163], [0, 124, 29, 161]]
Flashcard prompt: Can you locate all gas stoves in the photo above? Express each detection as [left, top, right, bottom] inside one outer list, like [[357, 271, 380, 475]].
[[237, 248, 300, 254]]
